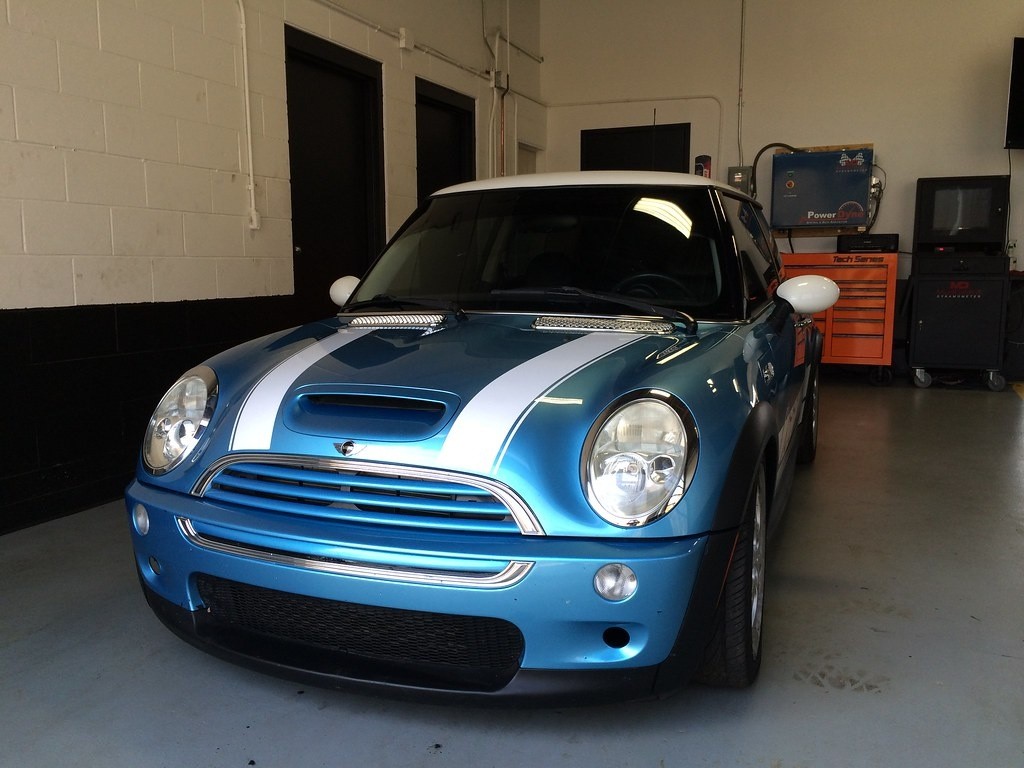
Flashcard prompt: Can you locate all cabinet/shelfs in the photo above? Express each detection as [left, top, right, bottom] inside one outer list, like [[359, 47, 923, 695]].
[[906, 250, 1011, 393], [778, 250, 898, 368]]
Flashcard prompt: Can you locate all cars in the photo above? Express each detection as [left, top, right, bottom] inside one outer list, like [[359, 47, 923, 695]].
[[123, 159, 847, 713]]
